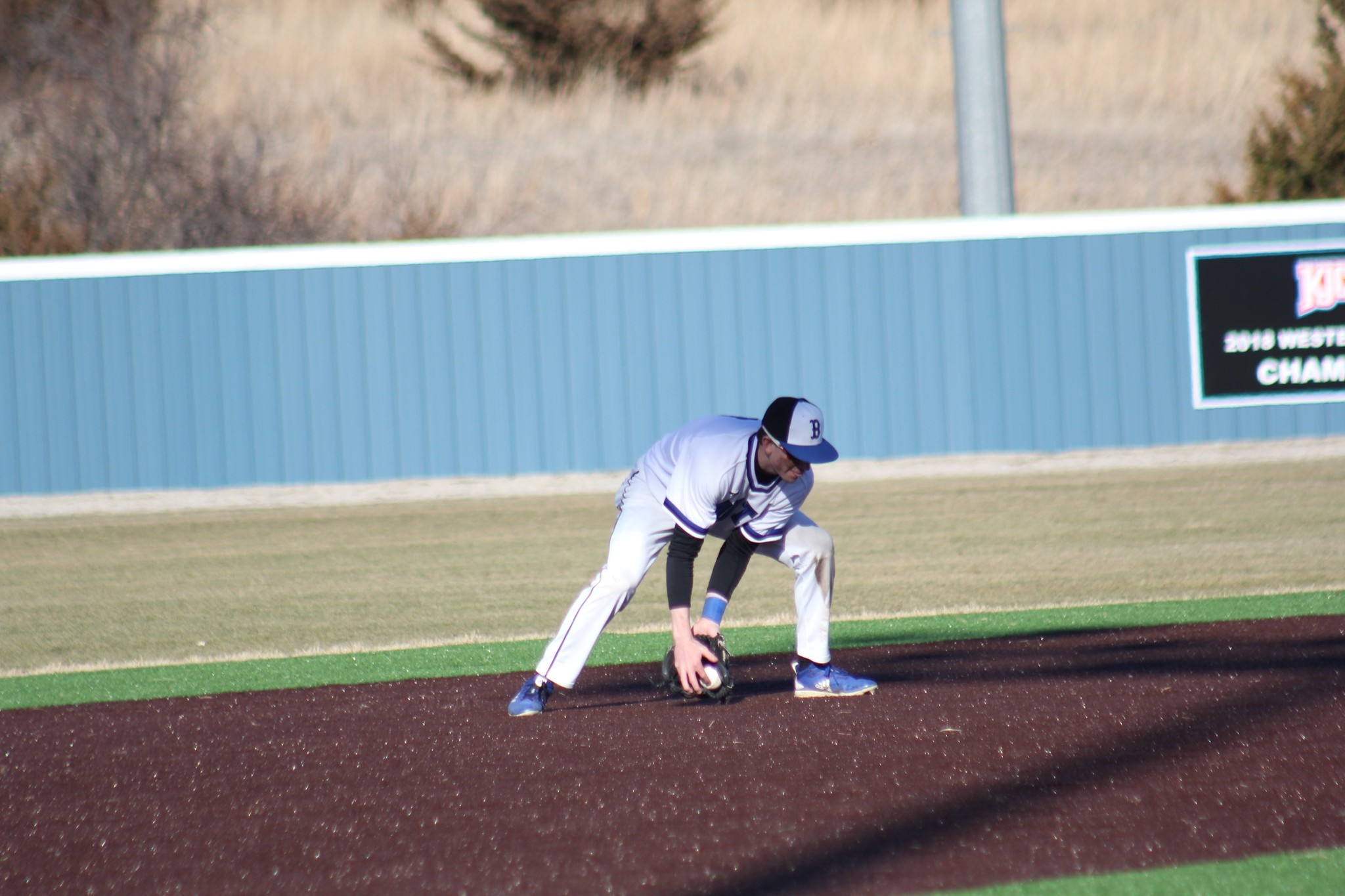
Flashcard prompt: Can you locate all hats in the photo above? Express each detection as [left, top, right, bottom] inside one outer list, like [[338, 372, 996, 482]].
[[761, 396, 838, 464]]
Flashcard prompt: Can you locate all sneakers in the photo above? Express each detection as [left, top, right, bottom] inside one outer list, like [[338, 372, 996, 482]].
[[791, 661, 878, 696], [507, 675, 554, 716]]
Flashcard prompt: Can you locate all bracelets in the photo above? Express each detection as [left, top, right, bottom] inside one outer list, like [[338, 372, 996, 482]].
[[701, 597, 728, 625]]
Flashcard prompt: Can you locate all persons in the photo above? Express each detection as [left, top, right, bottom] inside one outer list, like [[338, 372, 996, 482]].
[[508, 396, 880, 718]]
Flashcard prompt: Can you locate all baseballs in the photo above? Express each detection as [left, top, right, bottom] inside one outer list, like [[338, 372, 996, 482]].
[[700, 666, 722, 690]]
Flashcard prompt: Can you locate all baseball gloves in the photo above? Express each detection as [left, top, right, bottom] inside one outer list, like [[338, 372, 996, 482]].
[[662, 632, 736, 700]]
